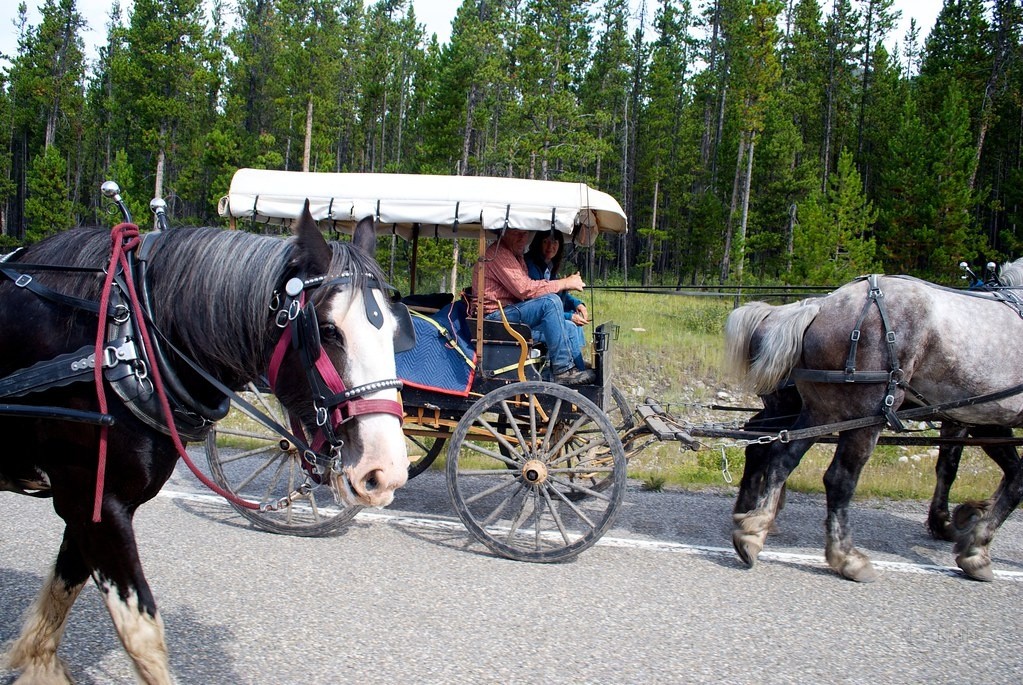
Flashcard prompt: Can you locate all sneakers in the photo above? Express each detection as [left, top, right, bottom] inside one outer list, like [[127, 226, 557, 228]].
[[554, 368, 595, 386]]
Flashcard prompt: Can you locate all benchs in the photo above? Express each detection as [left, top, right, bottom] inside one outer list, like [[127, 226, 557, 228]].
[[461, 289, 541, 382]]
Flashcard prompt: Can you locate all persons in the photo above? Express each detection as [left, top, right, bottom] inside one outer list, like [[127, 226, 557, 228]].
[[521, 229, 593, 369], [470, 228, 597, 384]]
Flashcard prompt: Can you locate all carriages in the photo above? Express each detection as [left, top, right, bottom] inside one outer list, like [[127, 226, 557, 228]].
[[201, 166, 1023, 585]]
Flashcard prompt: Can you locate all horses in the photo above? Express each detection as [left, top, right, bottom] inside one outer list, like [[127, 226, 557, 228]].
[[719, 256, 1023, 584], [1, 198, 408, 685]]
